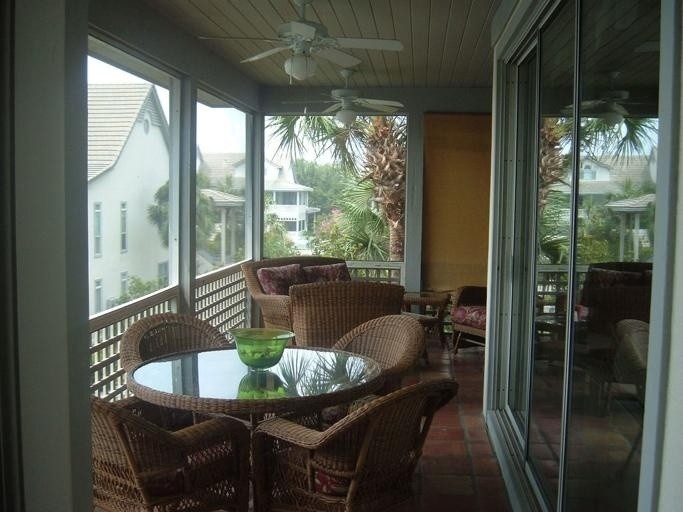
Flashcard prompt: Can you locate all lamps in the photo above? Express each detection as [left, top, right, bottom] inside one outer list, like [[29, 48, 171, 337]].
[[280, 44, 317, 81], [334, 102, 357, 131]]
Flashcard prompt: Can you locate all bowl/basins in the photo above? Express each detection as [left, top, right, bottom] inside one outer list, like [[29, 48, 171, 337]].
[[224, 327, 297, 370], [236, 370, 287, 401]]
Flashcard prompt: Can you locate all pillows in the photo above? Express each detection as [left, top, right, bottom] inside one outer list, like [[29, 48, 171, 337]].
[[258, 263, 298, 299], [302, 263, 346, 282], [450, 304, 487, 331], [584, 267, 642, 291]]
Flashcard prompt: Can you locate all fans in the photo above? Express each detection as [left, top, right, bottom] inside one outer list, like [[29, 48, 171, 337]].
[[197, 0, 402, 69], [281, 69, 403, 121]]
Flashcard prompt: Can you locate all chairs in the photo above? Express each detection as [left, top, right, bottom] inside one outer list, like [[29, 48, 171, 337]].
[[581, 261, 652, 324], [89, 397, 249, 510], [240, 256, 351, 348], [611, 318, 649, 481], [120, 312, 231, 372], [451, 285, 487, 353], [250, 375, 460, 512], [271, 313, 426, 428], [286, 282, 405, 349]]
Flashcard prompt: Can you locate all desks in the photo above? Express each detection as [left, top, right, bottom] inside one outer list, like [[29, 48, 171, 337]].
[[127, 342, 381, 427]]
